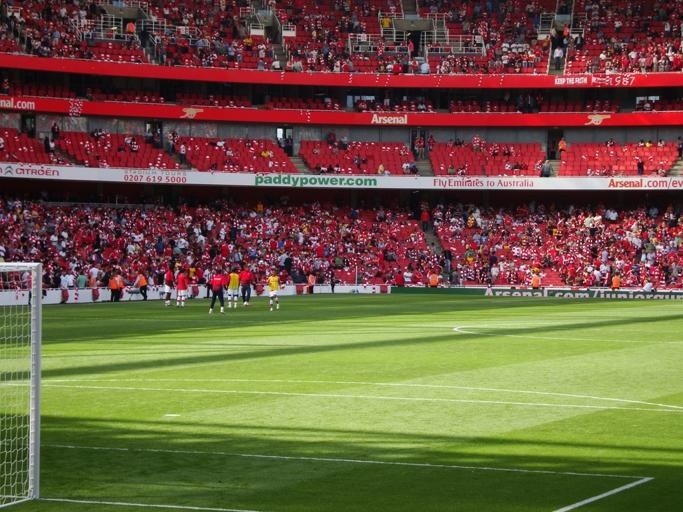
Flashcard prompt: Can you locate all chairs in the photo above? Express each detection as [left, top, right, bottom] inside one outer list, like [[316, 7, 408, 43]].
[[0, 0, 682, 305]]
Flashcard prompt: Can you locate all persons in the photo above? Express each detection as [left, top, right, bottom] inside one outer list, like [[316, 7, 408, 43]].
[[109, 264, 280, 315], [1, 1, 682, 298]]
